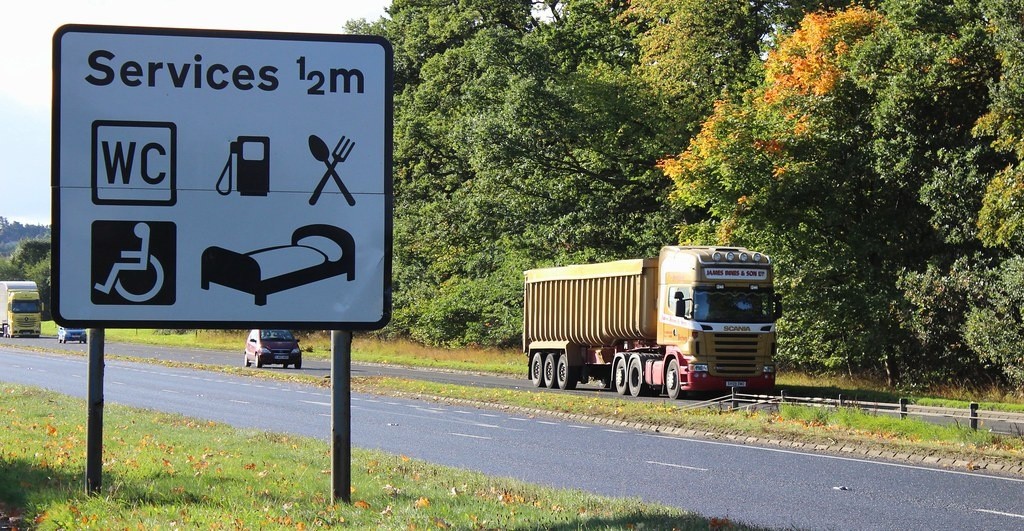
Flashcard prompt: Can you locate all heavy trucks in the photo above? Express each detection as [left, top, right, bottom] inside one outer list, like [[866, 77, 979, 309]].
[[521, 242, 784, 400], [1, 281, 44, 337]]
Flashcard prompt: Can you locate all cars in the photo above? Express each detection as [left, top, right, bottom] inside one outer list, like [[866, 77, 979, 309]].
[[244, 329, 303, 368], [58, 327, 87, 344]]
